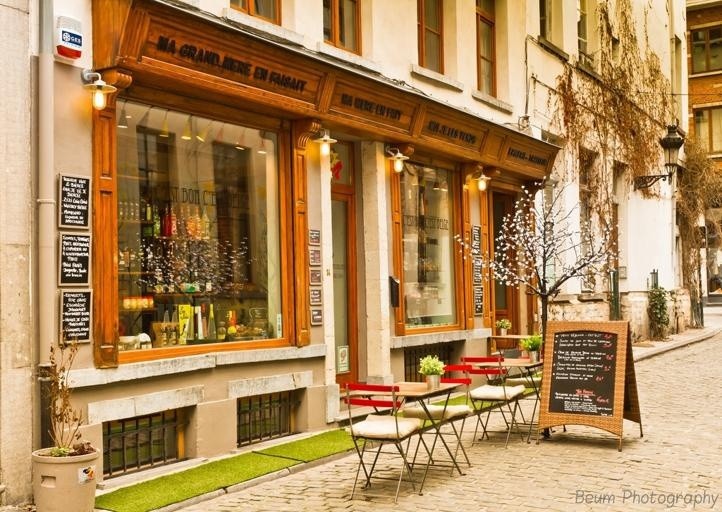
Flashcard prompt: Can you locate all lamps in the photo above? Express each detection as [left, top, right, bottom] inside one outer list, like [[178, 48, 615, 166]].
[[384, 143, 412, 174], [466, 160, 492, 193], [635, 120, 687, 191], [119, 97, 271, 154], [81, 66, 119, 113], [312, 124, 338, 158]]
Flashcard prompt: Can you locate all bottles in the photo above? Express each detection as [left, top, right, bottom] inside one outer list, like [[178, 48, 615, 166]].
[[202, 207, 210, 240], [170, 206, 177, 235], [185, 206, 193, 237], [152, 205, 161, 235], [144, 204, 152, 234], [177, 206, 185, 234], [161, 310, 170, 346], [162, 207, 171, 236], [201, 304, 208, 338], [170, 310, 179, 345], [208, 304, 217, 339], [193, 207, 201, 238]]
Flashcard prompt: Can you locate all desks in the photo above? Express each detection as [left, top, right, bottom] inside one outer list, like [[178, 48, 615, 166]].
[[488, 334, 543, 358]]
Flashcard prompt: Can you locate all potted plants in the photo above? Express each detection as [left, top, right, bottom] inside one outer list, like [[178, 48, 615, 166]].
[[496, 318, 513, 338], [30, 327, 102, 512], [520, 335, 545, 366], [418, 354, 448, 391]]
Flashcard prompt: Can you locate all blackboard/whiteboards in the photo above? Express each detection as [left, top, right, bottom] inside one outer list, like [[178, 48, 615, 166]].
[[310, 309, 323, 326], [58, 289, 93, 345], [309, 269, 322, 286], [538, 320, 640, 436], [308, 249, 322, 266], [308, 228, 321, 246], [473, 256, 483, 285], [473, 286, 484, 316], [471, 225, 482, 255], [310, 288, 322, 306], [58, 173, 93, 230], [57, 231, 92, 287]]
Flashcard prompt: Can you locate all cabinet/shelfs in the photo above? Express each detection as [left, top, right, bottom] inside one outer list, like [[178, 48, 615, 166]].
[[403, 236, 446, 301], [116, 173, 190, 339]]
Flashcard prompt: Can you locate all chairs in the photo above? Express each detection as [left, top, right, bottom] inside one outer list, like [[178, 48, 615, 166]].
[[335, 355, 543, 506]]
